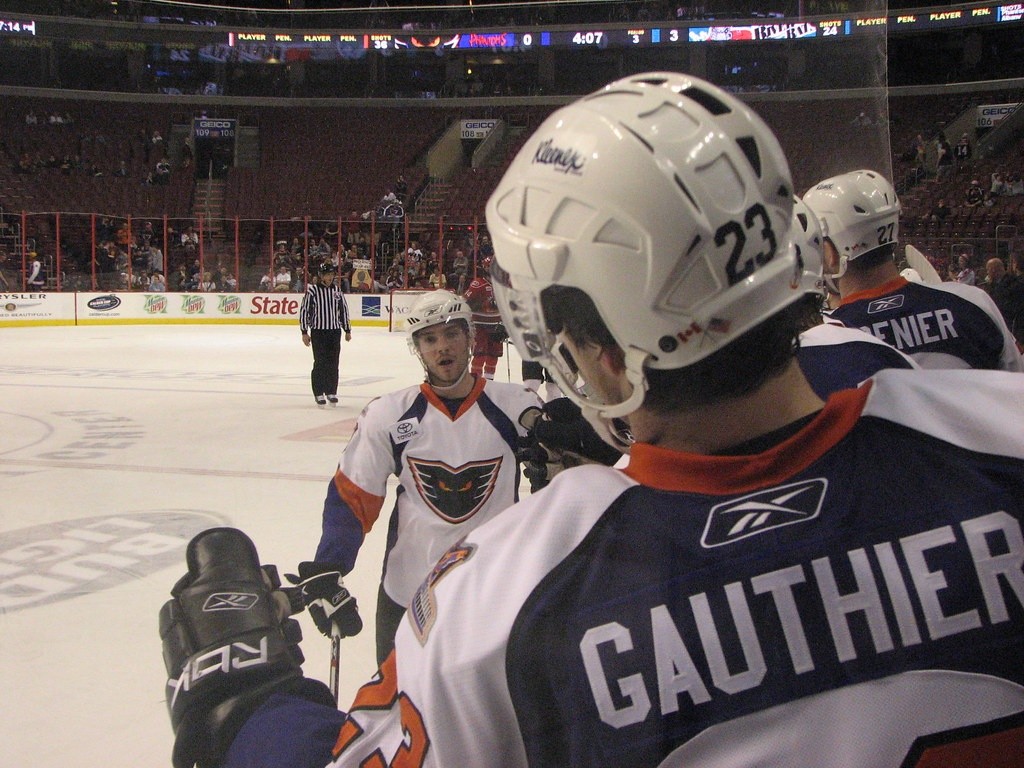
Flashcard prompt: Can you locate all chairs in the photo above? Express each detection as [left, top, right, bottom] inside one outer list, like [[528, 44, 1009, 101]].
[[892, 193, 1024, 266]]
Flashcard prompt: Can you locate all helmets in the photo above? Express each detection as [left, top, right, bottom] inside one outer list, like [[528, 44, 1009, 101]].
[[802, 168, 902, 264], [900, 268, 923, 283], [485, 69, 822, 372], [405, 291, 476, 353], [318, 263, 336, 277]]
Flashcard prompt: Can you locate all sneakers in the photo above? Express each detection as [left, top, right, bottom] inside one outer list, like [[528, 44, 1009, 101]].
[[327, 395, 338, 407], [315, 395, 326, 408]]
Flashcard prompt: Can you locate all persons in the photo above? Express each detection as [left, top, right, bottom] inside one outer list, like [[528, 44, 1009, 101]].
[[158, 68, 1024, 768], [299, 262, 351, 405], [0, 128, 237, 292], [250, 117, 1024, 495], [284, 290, 545, 675]]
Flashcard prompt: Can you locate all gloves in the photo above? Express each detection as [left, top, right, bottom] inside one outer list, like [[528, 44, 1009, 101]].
[[515, 399, 620, 493], [157, 526, 338, 768], [284, 561, 363, 640]]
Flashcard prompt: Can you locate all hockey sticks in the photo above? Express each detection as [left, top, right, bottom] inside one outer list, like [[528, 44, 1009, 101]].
[[506, 335, 510, 383], [502, 340, 514, 345], [904, 242, 943, 283], [328, 618, 342, 708]]
